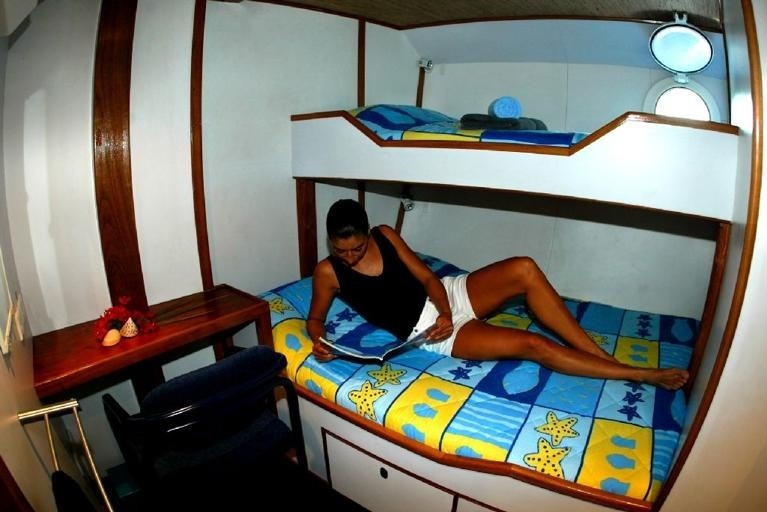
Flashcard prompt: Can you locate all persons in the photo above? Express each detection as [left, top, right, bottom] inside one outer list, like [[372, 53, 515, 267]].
[[306, 198, 691, 392]]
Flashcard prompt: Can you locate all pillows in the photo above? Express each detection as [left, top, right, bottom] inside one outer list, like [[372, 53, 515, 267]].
[[346, 104, 460, 131]]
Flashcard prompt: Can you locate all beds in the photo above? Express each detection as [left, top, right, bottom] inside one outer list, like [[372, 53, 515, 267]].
[[254, 0, 767, 512]]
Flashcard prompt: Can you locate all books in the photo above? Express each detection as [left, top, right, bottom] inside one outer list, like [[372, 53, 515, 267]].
[[317, 323, 437, 364]]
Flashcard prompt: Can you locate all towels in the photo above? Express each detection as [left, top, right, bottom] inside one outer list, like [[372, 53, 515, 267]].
[[488, 96, 522, 120], [51, 470, 97, 512], [460, 114, 547, 130]]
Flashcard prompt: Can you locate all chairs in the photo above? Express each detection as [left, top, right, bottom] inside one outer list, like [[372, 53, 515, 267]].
[[102, 346, 313, 512]]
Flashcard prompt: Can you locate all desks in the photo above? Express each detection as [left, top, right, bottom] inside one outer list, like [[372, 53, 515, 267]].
[[32, 284, 275, 408]]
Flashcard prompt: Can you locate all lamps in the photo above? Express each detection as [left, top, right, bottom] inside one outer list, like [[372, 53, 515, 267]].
[[418, 59, 433, 70]]
